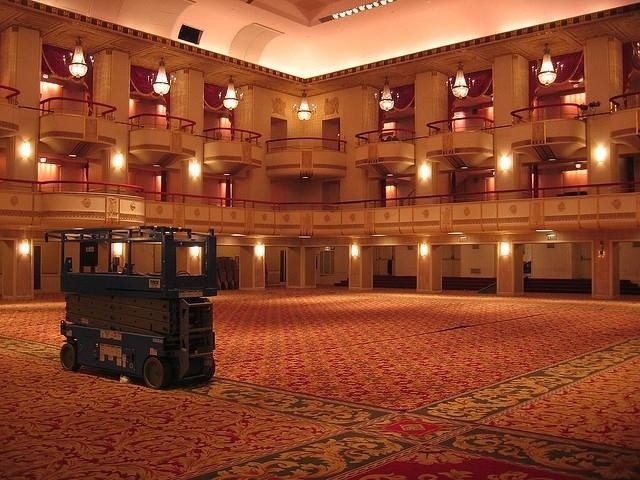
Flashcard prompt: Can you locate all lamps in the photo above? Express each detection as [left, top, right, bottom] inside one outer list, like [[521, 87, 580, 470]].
[[222, 76, 239, 111], [380, 81, 394, 112], [297, 90, 311, 121], [451, 61, 470, 100], [538, 44, 558, 86], [68, 37, 88, 79], [152, 59, 171, 97]]
